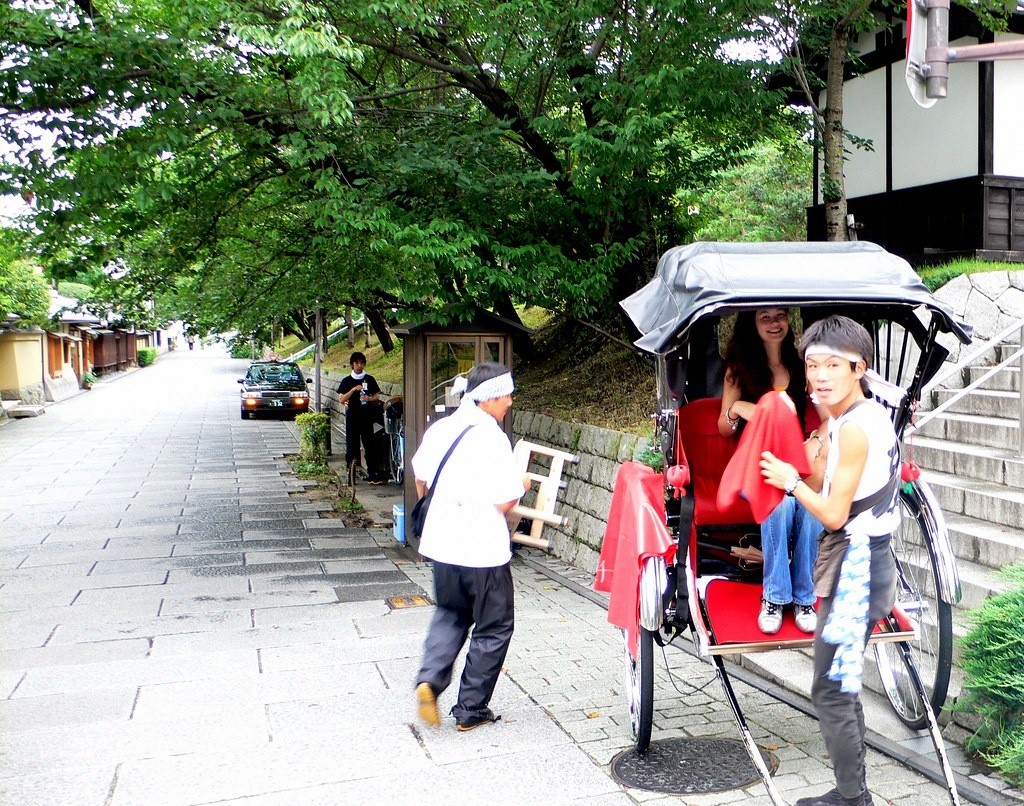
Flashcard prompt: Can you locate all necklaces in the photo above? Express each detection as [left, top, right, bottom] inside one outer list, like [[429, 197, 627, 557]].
[[770, 363, 782, 369]]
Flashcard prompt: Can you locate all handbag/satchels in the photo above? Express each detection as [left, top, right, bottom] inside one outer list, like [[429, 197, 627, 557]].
[[410, 495, 432, 537]]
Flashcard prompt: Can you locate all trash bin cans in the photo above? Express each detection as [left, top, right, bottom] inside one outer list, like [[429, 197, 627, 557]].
[[383, 394, 405, 484]]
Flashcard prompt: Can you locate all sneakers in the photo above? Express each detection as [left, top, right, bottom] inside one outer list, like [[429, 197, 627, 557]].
[[796, 786, 874, 806], [757, 599, 783, 633], [793, 603, 818, 633]]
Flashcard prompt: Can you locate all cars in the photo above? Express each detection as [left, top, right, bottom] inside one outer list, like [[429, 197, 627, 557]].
[[237, 360, 313, 418]]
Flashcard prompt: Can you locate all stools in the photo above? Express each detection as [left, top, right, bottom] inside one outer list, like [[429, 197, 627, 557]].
[[503, 437, 579, 548]]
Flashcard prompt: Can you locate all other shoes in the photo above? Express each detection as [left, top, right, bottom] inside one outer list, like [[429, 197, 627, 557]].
[[456, 711, 494, 730], [417, 682, 441, 727], [348, 469, 356, 486], [368, 475, 385, 485]]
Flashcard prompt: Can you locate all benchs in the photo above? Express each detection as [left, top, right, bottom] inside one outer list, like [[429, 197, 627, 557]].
[[679, 398, 820, 525]]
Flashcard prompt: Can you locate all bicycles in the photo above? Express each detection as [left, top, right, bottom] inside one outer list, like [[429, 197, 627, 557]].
[[383, 393, 406, 495]]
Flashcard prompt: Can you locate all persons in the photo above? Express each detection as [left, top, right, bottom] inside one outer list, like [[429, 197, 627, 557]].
[[716, 306, 820, 634], [188, 336, 195, 350], [411, 362, 533, 732], [336, 351, 386, 485], [757, 316, 902, 806]]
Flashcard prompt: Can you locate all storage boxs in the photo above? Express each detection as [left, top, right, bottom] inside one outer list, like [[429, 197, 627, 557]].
[[392, 503, 405, 542]]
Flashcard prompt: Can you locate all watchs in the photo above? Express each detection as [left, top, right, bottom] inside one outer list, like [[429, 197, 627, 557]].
[[784, 476, 803, 497]]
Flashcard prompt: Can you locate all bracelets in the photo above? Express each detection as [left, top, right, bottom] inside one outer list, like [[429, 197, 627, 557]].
[[725, 408, 740, 430], [813, 435, 825, 445]]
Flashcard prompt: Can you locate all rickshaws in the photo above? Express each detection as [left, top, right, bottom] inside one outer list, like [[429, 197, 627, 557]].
[[621, 240, 963, 806]]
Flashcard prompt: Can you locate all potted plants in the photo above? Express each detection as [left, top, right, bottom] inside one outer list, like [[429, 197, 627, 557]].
[[81, 371, 97, 390]]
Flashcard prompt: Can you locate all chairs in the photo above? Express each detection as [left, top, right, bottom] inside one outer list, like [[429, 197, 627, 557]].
[[256, 372, 269, 382], [279, 372, 292, 380]]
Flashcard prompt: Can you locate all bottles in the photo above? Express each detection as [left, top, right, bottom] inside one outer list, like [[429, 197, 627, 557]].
[[360, 389, 367, 405]]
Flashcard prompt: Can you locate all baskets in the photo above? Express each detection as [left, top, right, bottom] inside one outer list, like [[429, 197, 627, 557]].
[[383, 413, 400, 434]]
